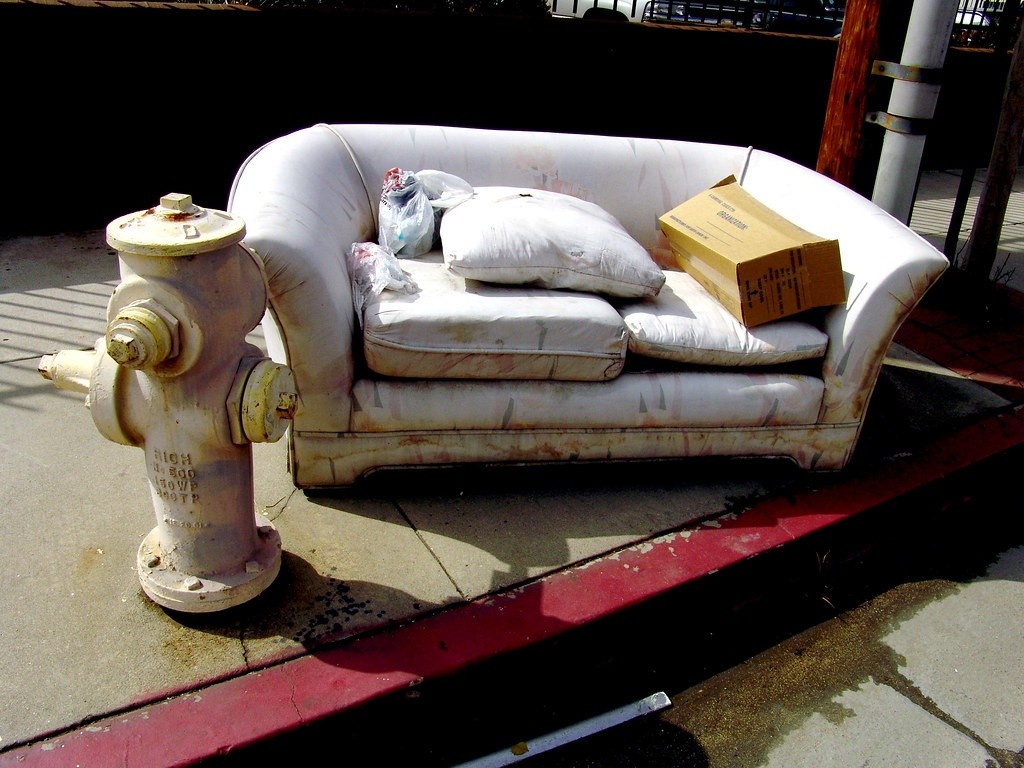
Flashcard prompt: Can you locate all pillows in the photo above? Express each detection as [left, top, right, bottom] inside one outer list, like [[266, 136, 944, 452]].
[[437, 186, 665, 301]]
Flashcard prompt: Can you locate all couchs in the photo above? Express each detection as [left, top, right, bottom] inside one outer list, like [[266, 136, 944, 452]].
[[227, 123, 950, 489]]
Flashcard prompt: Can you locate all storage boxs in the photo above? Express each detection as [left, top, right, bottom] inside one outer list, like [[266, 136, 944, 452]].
[[658, 174, 847, 329]]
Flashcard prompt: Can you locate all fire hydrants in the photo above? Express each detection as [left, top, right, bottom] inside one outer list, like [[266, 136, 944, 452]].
[[39, 192, 294, 613]]
